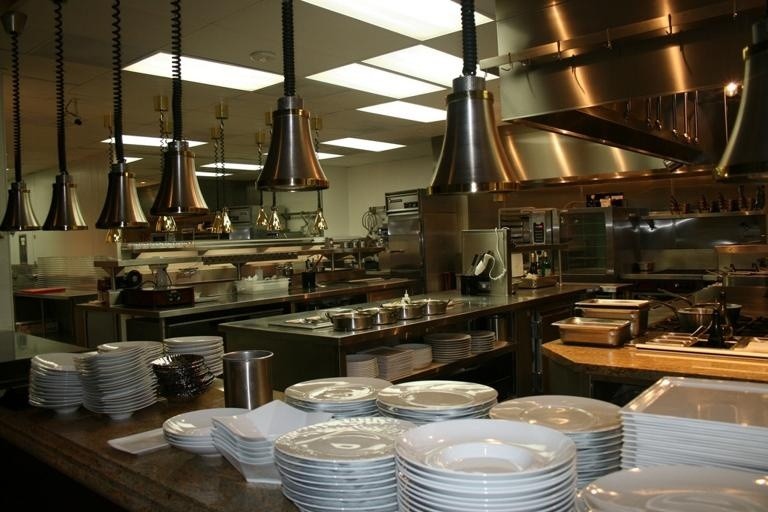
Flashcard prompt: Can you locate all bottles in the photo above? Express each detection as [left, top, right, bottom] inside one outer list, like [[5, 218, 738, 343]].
[[528, 251, 553, 279]]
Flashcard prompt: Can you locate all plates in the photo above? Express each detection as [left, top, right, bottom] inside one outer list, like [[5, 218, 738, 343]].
[[374, 379, 497, 426], [343, 324, 497, 385], [619, 368, 768, 476], [163, 406, 251, 461], [393, 418, 581, 512], [106, 424, 171, 457], [283, 375, 395, 421], [22, 332, 225, 422], [573, 464, 767, 512], [489, 394, 623, 497], [275, 416, 418, 512], [209, 397, 335, 486]]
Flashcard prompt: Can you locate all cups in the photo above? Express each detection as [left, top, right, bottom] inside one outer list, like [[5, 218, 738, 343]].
[[220, 350, 274, 412]]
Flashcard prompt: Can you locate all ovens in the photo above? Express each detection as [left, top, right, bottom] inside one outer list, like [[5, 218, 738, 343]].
[[384, 188, 422, 254]]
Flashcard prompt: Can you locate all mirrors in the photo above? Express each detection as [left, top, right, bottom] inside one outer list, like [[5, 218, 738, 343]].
[[122, 152, 323, 250]]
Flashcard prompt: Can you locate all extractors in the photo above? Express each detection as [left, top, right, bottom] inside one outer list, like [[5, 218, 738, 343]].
[[492, 1, 766, 188]]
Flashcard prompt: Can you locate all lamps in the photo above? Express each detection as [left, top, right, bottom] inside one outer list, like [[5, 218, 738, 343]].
[[428, 0, 527, 197], [94, 2, 151, 229], [0, 1, 41, 231], [149, 1, 209, 217], [253, 2, 330, 193], [40, 2, 91, 230]]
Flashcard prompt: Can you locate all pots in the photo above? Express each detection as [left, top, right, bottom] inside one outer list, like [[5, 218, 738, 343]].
[[638, 286, 744, 327], [322, 296, 451, 331]]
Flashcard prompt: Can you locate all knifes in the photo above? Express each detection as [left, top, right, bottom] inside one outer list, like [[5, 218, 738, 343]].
[[464, 249, 494, 276]]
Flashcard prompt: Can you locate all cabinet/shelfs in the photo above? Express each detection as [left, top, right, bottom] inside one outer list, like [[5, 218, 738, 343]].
[[522, 166, 768, 317]]
